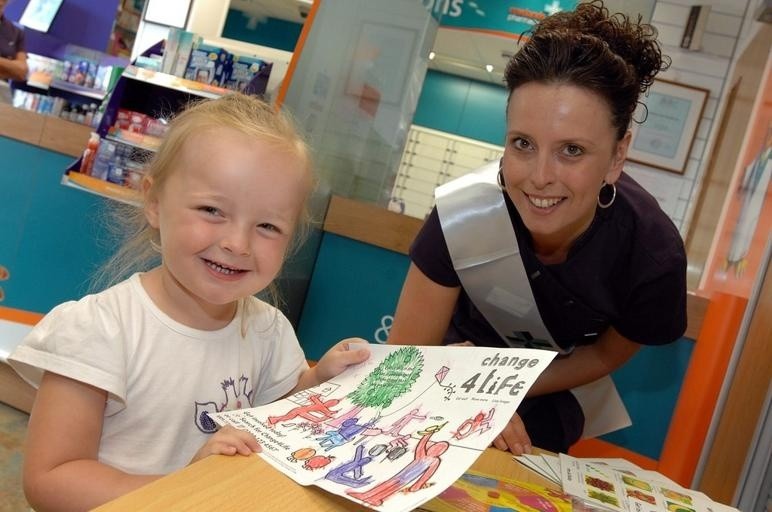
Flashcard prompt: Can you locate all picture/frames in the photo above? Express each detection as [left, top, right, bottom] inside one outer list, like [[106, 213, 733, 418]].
[[625, 76, 711, 175]]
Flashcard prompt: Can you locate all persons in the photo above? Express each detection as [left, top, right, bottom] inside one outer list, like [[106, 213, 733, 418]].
[[380, 0, 686, 457], [1, 0, 29, 82], [21, 93, 372, 512]]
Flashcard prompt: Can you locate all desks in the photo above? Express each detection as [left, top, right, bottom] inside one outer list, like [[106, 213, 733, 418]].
[[86, 444, 572, 512]]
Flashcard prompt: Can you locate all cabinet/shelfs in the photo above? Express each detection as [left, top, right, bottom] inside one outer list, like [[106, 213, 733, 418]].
[[58, 37, 274, 209], [0, 46, 129, 129]]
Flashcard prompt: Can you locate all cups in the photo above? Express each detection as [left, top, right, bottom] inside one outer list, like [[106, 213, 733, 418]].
[[26, 53, 57, 91]]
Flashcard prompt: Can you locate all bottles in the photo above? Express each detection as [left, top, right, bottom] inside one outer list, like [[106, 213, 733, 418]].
[[58, 101, 104, 129]]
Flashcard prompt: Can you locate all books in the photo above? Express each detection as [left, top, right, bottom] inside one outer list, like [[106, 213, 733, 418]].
[[513, 452, 741, 512]]
